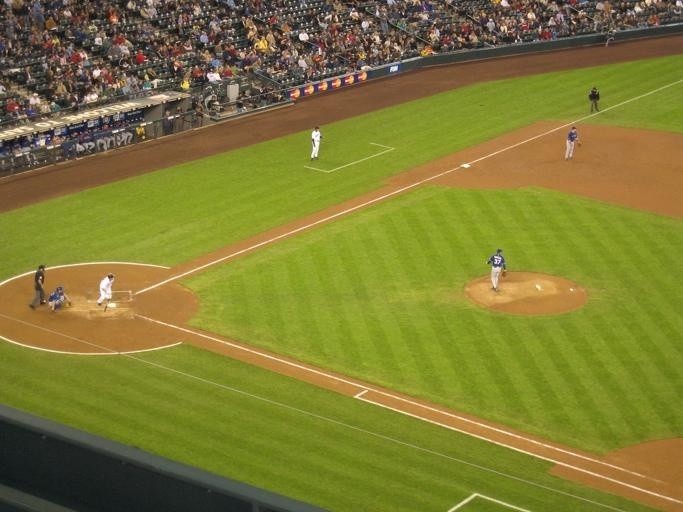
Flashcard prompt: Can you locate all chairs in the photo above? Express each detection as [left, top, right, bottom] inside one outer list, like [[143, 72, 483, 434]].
[[1, 1, 358, 128], [222, 0, 682, 70]]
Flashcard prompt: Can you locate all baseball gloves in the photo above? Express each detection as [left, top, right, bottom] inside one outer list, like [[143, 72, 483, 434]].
[[501, 270, 506, 277], [577, 140, 582, 145], [68, 301, 71, 305]]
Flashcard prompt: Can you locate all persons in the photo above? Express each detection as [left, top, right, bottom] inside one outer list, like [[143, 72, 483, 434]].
[[311, 127, 322, 160], [565, 127, 580, 160], [97, 272, 116, 312], [298, 1, 682, 86], [588, 87, 600, 112], [29, 265, 47, 309], [486, 248, 507, 291], [47, 287, 71, 313], [1, 1, 298, 174]]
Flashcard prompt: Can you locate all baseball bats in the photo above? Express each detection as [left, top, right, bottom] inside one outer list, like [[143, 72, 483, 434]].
[[104, 293, 110, 312]]
